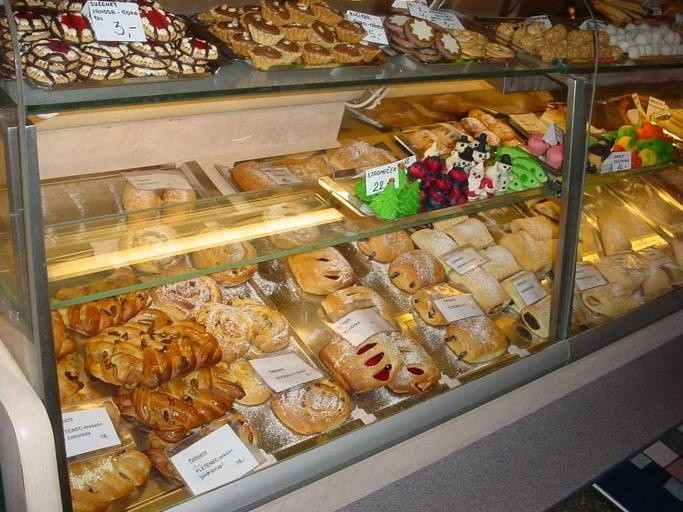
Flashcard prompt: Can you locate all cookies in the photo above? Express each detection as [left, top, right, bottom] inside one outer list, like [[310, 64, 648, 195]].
[[384, 12, 515, 63], [0, 1, 219, 88]]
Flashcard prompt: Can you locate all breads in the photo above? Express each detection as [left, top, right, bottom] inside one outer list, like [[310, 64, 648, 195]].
[[198, 0, 391, 73], [411, 282, 461, 324], [232, 144, 395, 191], [272, 376, 350, 433], [500, 165, 683, 319], [222, 360, 271, 406], [122, 182, 165, 225], [387, 250, 446, 294], [160, 186, 199, 224], [476, 244, 521, 282], [195, 304, 254, 362], [321, 284, 386, 321], [118, 223, 185, 274], [264, 202, 322, 250], [320, 328, 405, 397], [384, 333, 442, 398], [289, 246, 354, 296], [528, 110, 683, 181], [404, 109, 518, 161], [150, 277, 222, 325], [245, 307, 290, 352], [358, 230, 415, 265], [446, 216, 495, 253], [444, 316, 507, 362], [50, 310, 247, 512], [522, 294, 554, 338], [423, 204, 468, 232], [52, 265, 154, 336], [187, 240, 258, 287], [511, 313, 543, 345]]
